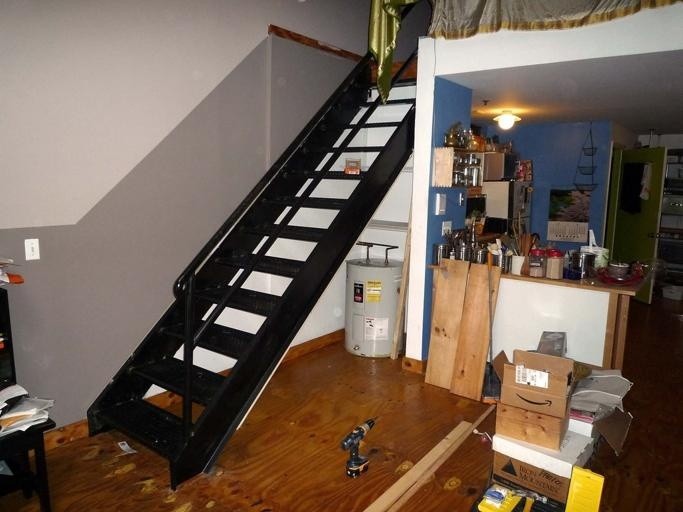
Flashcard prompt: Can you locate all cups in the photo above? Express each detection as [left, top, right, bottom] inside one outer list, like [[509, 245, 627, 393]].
[[491, 254, 511, 275], [607, 261, 631, 280]]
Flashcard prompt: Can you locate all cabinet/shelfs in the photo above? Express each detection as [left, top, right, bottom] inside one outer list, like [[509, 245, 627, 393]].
[[432, 146, 484, 188]]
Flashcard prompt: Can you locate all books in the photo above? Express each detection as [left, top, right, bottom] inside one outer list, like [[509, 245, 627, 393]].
[[0, 384, 55, 437]]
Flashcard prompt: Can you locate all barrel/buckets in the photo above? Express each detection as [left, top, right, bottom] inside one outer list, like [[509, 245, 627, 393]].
[[579, 229, 609, 268], [345, 258, 403, 358]]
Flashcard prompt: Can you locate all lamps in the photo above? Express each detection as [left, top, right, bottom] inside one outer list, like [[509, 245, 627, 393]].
[[494, 110, 521, 131]]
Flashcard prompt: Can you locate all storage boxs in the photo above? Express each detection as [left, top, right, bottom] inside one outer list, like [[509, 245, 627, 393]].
[[491, 349, 633, 504]]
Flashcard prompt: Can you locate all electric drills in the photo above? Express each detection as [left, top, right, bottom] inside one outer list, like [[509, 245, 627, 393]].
[[341, 415, 379, 477]]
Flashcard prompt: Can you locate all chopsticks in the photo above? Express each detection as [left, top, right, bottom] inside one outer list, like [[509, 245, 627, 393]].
[[510, 210, 534, 256]]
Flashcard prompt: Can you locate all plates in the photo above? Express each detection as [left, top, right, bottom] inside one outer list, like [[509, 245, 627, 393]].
[[596, 266, 644, 286]]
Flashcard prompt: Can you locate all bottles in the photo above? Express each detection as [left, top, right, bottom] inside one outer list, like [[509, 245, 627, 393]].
[[433, 242, 451, 266], [546, 248, 564, 280], [571, 251, 596, 279], [528, 248, 546, 277], [455, 246, 472, 262], [474, 247, 488, 263]]
